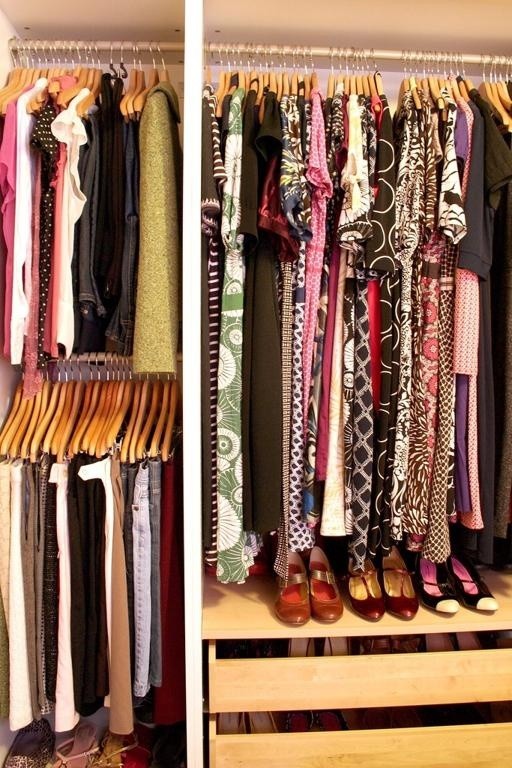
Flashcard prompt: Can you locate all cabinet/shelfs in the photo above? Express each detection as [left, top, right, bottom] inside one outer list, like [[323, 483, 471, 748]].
[[0, 2, 512, 768]]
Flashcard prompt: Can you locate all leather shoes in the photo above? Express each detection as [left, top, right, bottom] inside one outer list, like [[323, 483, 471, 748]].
[[412, 555, 461, 614], [445, 552, 499, 611], [275, 547, 311, 624], [308, 546, 344, 624], [379, 544, 420, 620], [225, 633, 482, 662], [217, 708, 422, 734], [346, 548, 386, 622]]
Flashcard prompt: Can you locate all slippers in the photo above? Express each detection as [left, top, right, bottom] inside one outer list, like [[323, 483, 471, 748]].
[[53, 721, 100, 767]]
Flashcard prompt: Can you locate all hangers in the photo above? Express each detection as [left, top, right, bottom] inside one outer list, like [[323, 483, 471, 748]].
[[0, 34, 512, 136], [1, 351, 178, 464]]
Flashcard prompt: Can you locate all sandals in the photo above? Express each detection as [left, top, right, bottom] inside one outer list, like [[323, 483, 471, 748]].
[[92, 726, 186, 768]]
